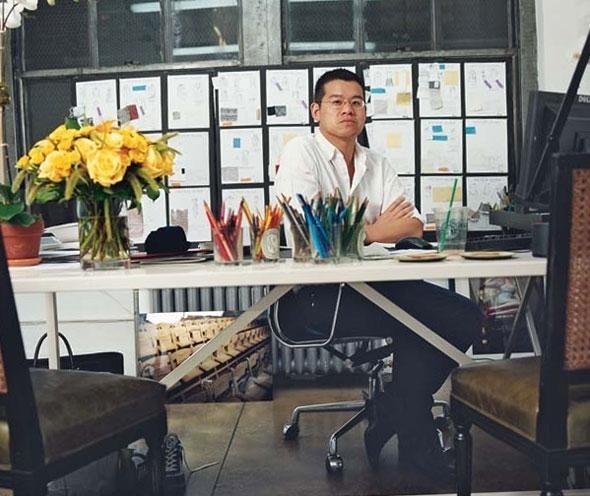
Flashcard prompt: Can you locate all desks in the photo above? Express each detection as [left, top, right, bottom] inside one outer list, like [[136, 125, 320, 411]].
[[9, 249, 548, 392]]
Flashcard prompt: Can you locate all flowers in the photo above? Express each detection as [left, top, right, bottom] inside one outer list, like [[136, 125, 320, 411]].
[[11, 114, 182, 261]]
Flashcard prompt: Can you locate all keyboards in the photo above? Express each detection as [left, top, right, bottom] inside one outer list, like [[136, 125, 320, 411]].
[[442, 233, 537, 252]]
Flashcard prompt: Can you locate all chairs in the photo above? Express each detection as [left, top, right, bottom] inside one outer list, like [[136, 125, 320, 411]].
[[0, 231, 168, 496], [450, 151, 590, 496], [139, 318, 271, 401], [268, 224, 455, 472]]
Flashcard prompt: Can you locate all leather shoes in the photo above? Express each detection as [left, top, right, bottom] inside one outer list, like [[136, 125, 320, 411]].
[[364, 397, 396, 468], [397, 436, 455, 486]]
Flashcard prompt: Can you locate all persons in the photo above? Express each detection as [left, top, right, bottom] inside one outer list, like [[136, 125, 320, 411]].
[[273, 67, 487, 476]]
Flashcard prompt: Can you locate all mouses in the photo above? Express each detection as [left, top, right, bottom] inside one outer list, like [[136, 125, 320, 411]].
[[394, 236, 433, 250]]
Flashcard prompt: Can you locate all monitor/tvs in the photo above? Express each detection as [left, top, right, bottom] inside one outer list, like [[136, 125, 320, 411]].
[[514, 92, 590, 209]]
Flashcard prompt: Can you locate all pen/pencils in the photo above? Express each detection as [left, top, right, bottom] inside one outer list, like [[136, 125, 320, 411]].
[[203, 199, 243, 263], [276, 187, 369, 260], [241, 197, 292, 260]]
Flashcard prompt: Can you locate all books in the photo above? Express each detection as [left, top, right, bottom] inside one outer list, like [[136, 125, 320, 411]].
[[139, 255, 214, 264], [39, 221, 139, 258]]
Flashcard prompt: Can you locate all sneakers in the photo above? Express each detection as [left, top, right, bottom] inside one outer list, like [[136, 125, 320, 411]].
[[160, 437, 187, 495]]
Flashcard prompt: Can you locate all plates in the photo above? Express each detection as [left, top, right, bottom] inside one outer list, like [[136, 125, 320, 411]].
[[459, 250, 516, 259], [393, 253, 448, 262]]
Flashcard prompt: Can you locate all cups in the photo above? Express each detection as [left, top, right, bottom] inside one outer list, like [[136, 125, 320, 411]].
[[289, 224, 367, 265], [530, 222, 548, 258], [431, 206, 468, 260], [211, 226, 243, 266], [248, 224, 281, 263]]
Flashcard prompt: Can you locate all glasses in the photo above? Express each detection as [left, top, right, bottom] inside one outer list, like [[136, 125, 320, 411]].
[[318, 97, 364, 108]]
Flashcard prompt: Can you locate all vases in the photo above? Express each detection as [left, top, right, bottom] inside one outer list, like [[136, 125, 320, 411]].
[[77, 194, 130, 272]]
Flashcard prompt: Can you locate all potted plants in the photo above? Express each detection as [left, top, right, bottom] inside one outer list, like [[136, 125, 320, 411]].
[[0, 184, 44, 266]]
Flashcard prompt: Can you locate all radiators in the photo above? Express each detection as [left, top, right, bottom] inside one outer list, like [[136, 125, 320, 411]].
[[152, 285, 382, 376]]
